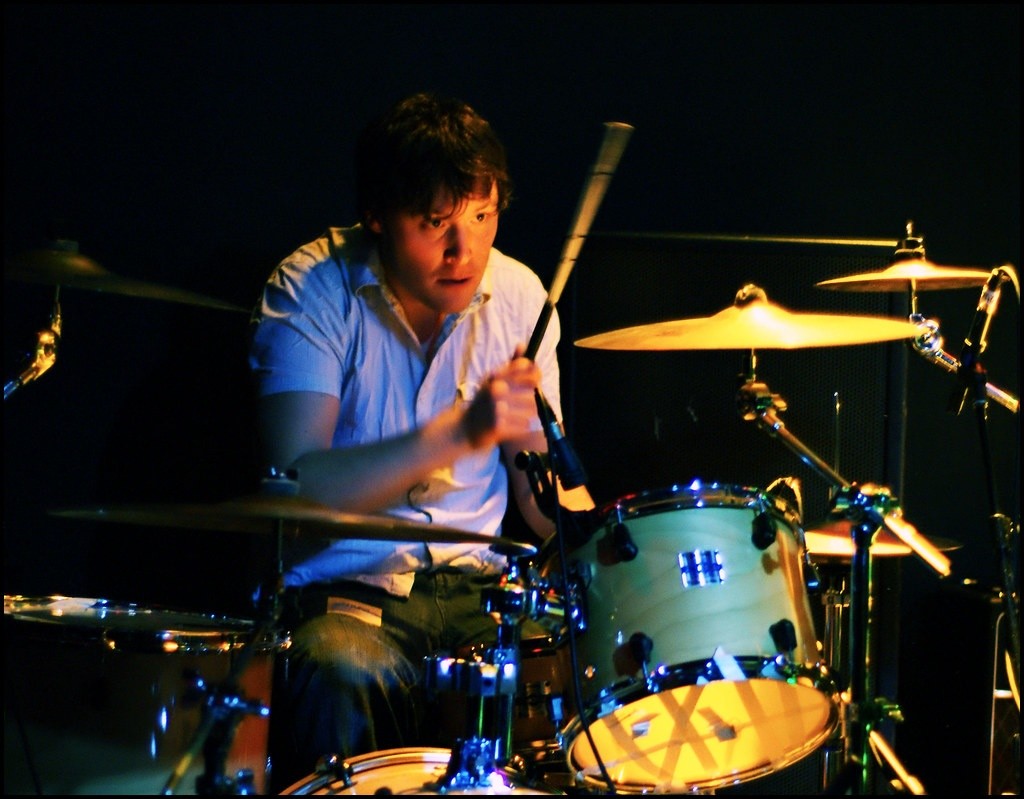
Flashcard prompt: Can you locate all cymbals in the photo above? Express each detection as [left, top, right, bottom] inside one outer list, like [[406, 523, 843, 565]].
[[571, 288, 934, 353], [796, 516, 964, 559], [815, 235, 1011, 292]]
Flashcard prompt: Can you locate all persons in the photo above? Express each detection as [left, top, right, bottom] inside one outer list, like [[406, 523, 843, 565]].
[[246, 92, 565, 795]]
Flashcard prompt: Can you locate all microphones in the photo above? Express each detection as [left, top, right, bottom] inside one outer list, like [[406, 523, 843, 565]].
[[945, 266, 1006, 417], [532, 386, 587, 492]]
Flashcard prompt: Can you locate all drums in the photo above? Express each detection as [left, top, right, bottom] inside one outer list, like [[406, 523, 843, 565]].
[[189, 578, 519, 799], [1, 595, 292, 798], [533, 479, 841, 796]]
